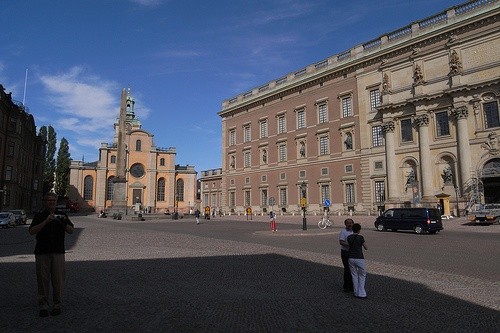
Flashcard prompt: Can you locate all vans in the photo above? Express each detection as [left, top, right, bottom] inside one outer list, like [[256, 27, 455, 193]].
[[375, 208, 444, 234]]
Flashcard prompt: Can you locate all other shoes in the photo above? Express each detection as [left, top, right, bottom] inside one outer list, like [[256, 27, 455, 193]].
[[52, 306, 62, 316], [39, 309, 49, 317]]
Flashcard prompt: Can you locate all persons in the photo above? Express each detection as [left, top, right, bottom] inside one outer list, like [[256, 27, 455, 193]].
[[337, 218, 355, 293], [270, 211, 277, 232], [195, 208, 201, 224], [29, 193, 75, 317], [345, 222, 368, 299]]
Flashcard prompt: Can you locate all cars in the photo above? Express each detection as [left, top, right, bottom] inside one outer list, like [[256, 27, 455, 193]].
[[7, 209, 27, 225], [0, 212, 16, 229]]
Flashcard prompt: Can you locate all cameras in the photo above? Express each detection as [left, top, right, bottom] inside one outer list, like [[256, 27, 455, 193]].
[[54, 215, 65, 219]]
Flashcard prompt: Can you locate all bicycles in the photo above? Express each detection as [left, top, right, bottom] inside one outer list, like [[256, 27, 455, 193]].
[[318, 217, 335, 230]]
